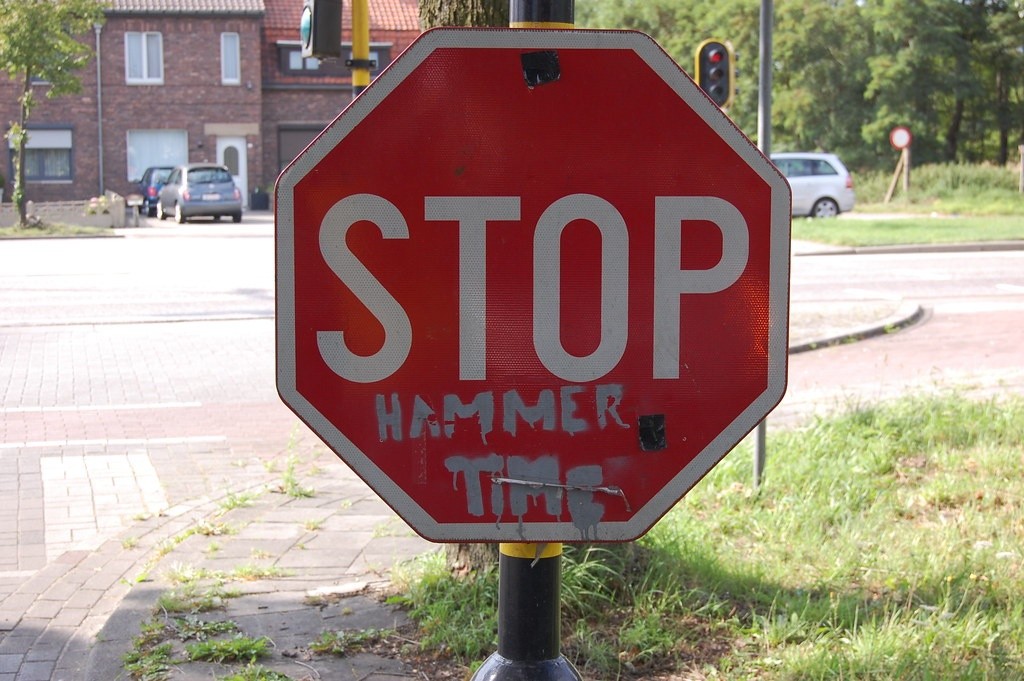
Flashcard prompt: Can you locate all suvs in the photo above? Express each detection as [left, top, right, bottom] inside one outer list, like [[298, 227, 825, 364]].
[[770, 153, 854, 217]]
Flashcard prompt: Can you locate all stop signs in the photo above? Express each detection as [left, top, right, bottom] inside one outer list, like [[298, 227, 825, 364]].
[[274, 29, 791, 539]]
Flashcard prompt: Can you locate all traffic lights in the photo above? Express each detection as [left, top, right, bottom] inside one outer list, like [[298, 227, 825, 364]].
[[300, 0, 343, 61], [695, 39, 731, 107]]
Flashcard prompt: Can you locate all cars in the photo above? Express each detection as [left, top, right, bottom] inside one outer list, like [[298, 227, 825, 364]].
[[157, 163, 243, 224], [137, 167, 176, 218]]
